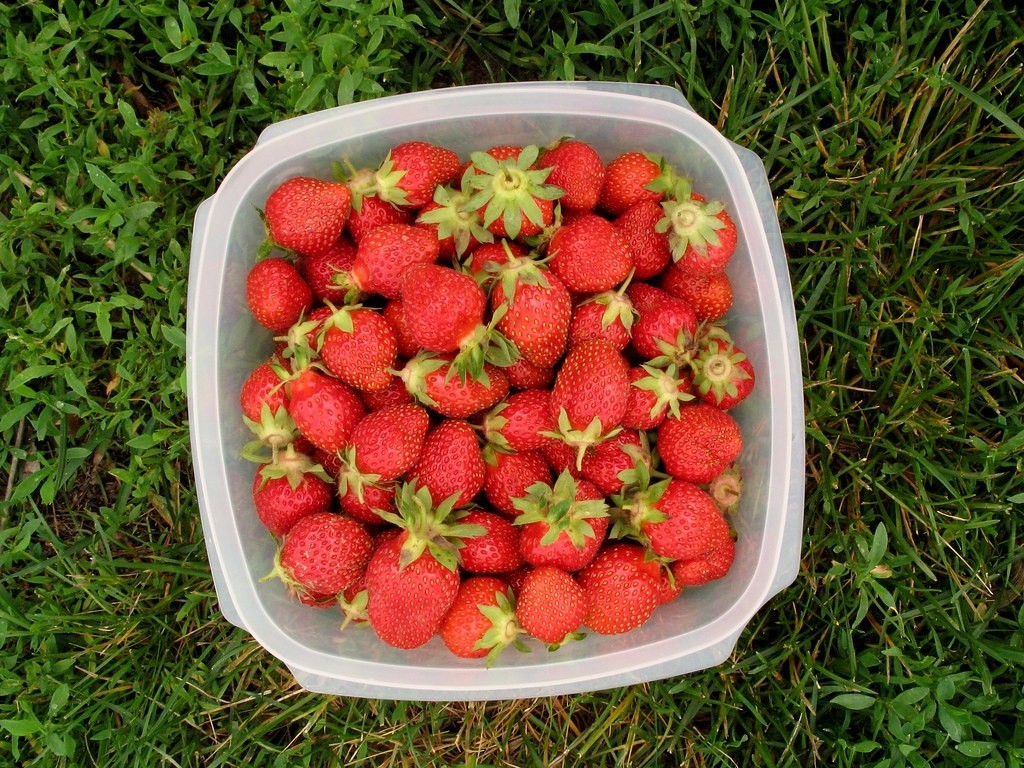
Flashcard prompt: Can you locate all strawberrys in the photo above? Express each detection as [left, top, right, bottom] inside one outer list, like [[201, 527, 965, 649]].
[[240, 135, 756, 667]]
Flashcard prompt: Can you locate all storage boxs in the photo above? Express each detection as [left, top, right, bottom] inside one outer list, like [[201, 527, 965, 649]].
[[186, 81, 805, 703]]
